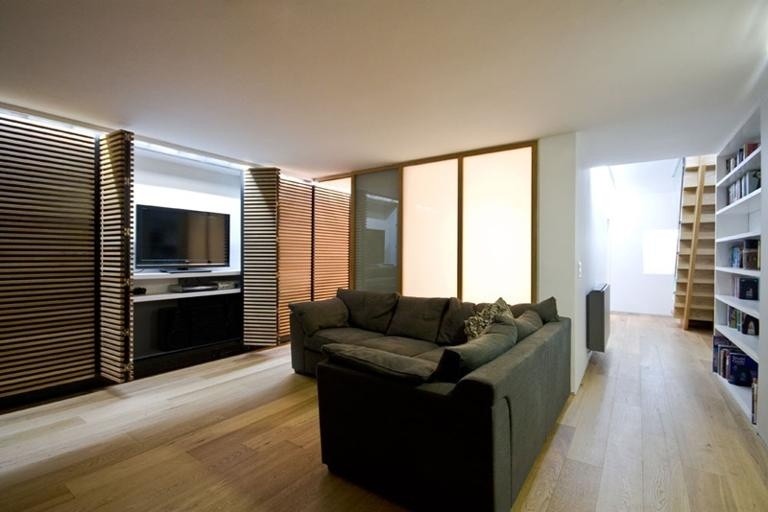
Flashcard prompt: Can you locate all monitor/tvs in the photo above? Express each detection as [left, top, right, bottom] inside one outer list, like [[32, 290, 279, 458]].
[[134, 204, 231, 274]]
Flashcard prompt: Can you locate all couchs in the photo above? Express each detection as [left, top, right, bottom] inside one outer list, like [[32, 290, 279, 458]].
[[286, 284, 571, 511]]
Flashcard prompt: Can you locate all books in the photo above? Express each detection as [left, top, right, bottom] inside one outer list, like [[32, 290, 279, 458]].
[[727, 138, 760, 206], [713, 240, 761, 424]]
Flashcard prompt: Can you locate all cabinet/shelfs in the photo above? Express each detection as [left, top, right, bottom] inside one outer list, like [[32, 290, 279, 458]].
[[672, 156, 714, 330], [713, 106, 764, 431]]
